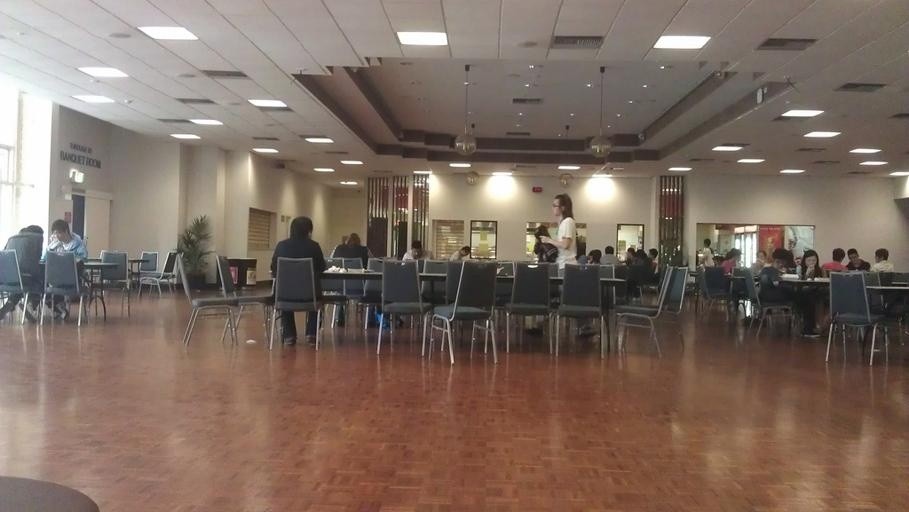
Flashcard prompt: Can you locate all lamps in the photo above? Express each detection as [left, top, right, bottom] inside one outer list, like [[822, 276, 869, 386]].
[[67, 163, 85, 186], [451, 63, 480, 158], [584, 65, 615, 157]]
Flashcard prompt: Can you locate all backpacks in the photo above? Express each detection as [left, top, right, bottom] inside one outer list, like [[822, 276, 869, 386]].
[[533, 226, 559, 263]]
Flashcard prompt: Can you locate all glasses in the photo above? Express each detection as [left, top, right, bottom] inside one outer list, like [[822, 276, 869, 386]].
[[552, 203, 561, 208]]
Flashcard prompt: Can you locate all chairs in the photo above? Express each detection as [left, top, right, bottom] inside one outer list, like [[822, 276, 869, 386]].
[[649, 267, 688, 353], [216, 255, 275, 346], [692, 259, 908, 369], [616, 266, 673, 357], [270, 257, 662, 364], [178, 254, 238, 346], [1, 246, 181, 331]]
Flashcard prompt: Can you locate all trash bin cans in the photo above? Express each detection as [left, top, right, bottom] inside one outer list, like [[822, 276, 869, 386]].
[[228, 259, 257, 287]]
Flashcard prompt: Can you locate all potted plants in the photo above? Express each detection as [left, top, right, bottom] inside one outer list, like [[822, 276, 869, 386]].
[[177, 212, 216, 291]]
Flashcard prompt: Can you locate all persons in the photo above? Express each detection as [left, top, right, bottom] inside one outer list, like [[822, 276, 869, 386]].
[[330, 233, 374, 326], [450, 247, 470, 261], [41, 220, 88, 320], [270, 216, 325, 344], [0, 226, 43, 322], [525, 195, 597, 338], [401, 241, 434, 266]]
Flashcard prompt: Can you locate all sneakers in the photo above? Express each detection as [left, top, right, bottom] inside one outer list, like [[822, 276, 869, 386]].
[[306, 336, 321, 347], [18, 302, 39, 323], [801, 329, 821, 339], [0, 300, 14, 320], [48, 304, 69, 319], [284, 335, 297, 345]]
